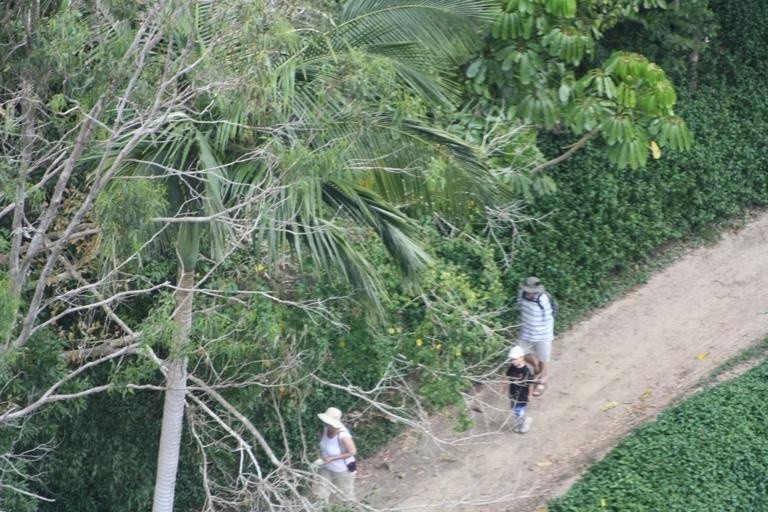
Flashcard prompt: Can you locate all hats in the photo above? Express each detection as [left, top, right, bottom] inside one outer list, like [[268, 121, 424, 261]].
[[520, 276, 545, 294], [507, 345, 525, 359], [317, 407, 344, 429]]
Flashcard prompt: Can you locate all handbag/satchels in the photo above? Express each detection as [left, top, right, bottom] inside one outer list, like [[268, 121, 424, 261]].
[[347, 462, 356, 472]]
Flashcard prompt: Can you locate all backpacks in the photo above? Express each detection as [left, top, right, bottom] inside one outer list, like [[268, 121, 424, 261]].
[[521, 290, 559, 321]]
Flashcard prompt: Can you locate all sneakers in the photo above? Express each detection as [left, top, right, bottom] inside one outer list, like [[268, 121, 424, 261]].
[[512, 416, 532, 433]]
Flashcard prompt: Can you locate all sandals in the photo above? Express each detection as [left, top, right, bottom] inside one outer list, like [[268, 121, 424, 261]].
[[532, 368, 548, 397]]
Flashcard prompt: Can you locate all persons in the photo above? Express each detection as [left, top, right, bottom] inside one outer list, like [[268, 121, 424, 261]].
[[313, 407, 357, 510], [503, 345, 534, 434], [517, 277, 555, 398]]
